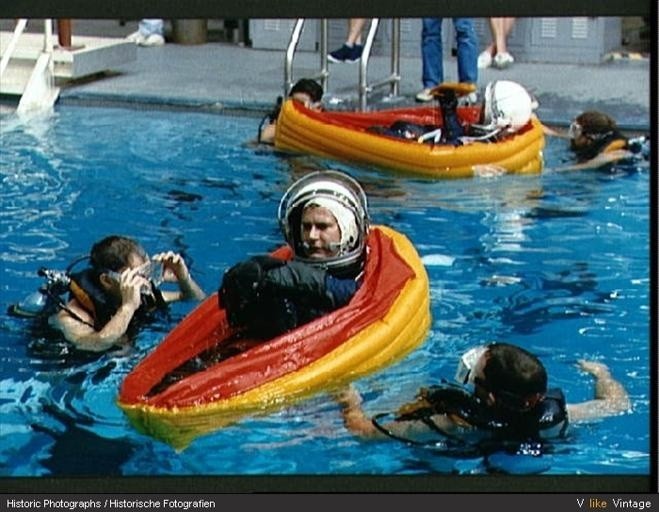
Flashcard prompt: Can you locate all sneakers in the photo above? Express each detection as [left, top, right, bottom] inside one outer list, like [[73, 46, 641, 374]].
[[477, 51, 514, 68], [327, 43, 363, 63], [416, 88, 434, 101]]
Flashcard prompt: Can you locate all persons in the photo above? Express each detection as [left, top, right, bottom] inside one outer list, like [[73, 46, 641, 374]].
[[120, 18, 167, 48], [26, 229, 205, 370], [146, 170, 365, 404], [320, 19, 365, 67], [359, 78, 534, 151], [251, 76, 329, 152], [533, 106, 642, 179], [326, 333, 636, 453], [474, 19, 520, 68], [414, 17, 478, 107]]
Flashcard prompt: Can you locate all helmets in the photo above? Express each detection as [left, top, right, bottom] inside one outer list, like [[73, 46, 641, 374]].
[[455, 343, 546, 407], [278, 170, 368, 270], [484, 81, 531, 134]]
